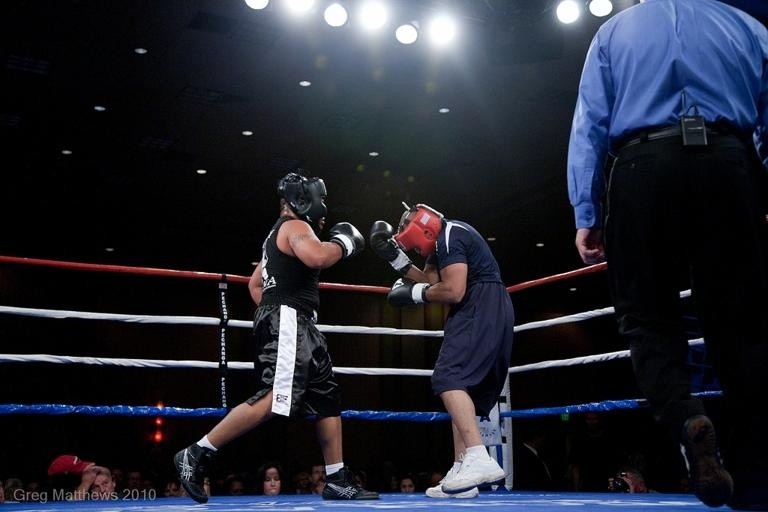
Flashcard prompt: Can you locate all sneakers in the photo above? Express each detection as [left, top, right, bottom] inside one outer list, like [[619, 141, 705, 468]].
[[425, 465, 479, 498], [679, 413, 734, 507], [323, 466, 379, 500], [442, 452, 506, 494], [173, 442, 222, 503]]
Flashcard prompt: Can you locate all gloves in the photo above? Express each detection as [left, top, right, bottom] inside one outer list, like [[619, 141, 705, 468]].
[[329, 221, 366, 262], [369, 220, 413, 274], [387, 277, 431, 306]]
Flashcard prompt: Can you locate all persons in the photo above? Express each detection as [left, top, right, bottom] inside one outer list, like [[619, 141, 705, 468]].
[[1, 408, 731, 504], [562, 0, 768, 512], [177, 166, 382, 504], [367, 202, 515, 504]]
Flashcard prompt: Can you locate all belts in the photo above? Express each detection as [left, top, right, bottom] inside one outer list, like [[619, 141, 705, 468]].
[[616, 125, 722, 151]]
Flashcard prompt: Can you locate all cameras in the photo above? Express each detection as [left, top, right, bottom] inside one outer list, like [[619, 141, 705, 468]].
[[612, 471, 631, 492]]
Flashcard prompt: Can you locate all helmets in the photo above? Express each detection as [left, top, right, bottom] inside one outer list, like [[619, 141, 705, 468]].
[[276, 177, 328, 234], [395, 203, 442, 258]]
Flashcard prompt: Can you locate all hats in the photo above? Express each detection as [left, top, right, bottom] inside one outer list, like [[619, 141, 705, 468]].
[[47, 454, 96, 483]]
